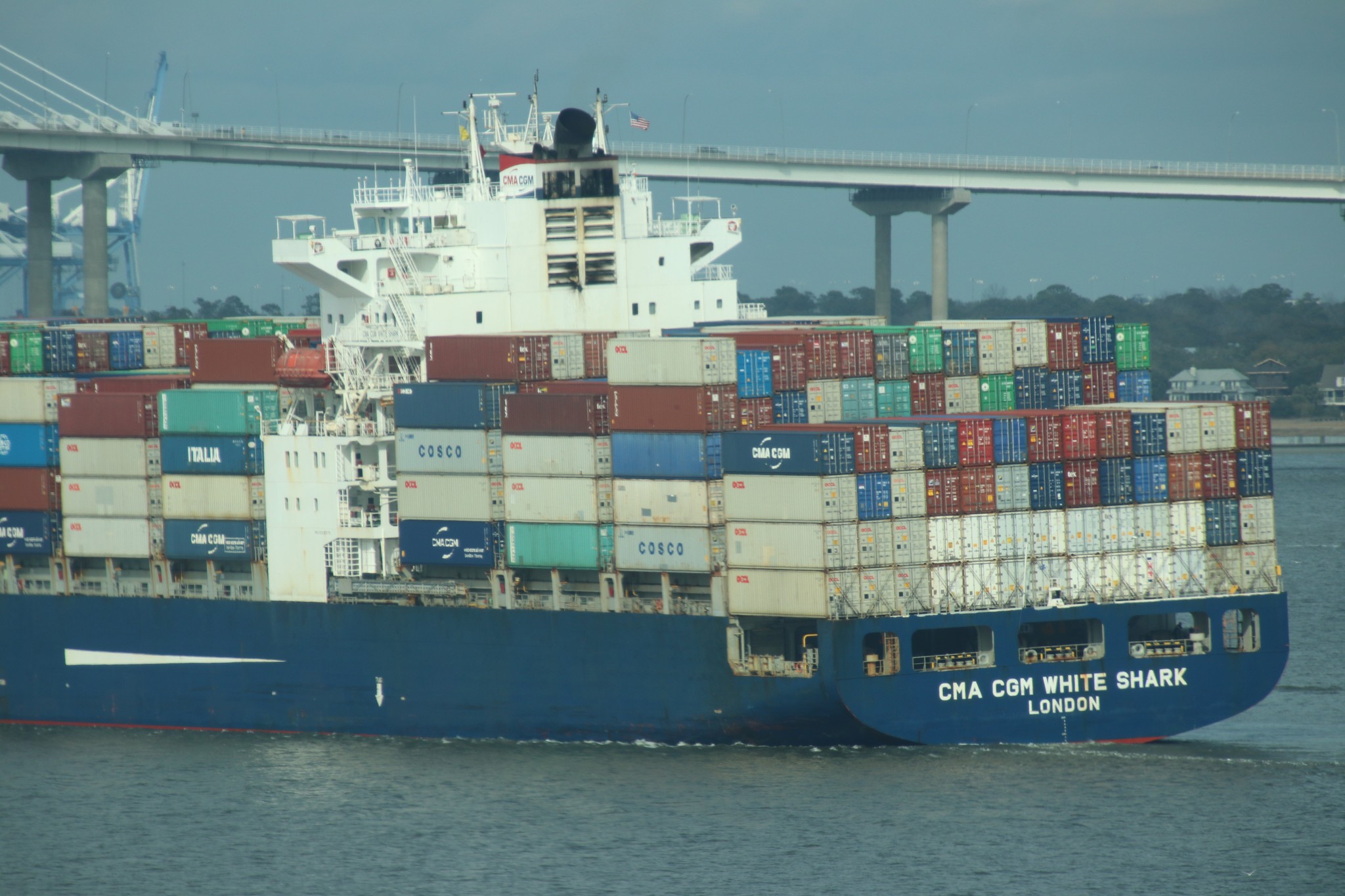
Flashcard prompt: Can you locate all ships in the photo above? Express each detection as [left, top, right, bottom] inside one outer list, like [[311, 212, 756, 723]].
[[0, 66, 1294, 745]]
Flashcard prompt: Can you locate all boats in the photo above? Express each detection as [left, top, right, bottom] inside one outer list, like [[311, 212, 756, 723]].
[[272, 345, 336, 389]]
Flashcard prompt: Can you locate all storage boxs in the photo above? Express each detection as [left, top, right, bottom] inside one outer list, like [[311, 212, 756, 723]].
[[0, 306, 1283, 622]]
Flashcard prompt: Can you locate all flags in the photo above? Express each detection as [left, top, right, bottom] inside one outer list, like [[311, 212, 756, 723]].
[[459, 125, 470, 141], [630, 110, 650, 131]]
